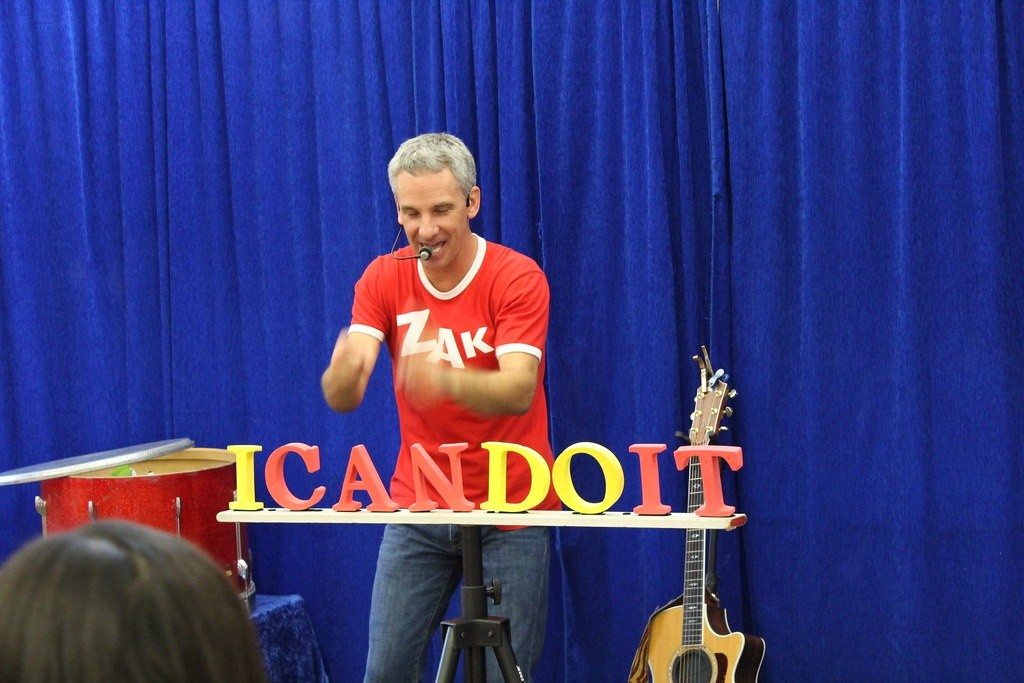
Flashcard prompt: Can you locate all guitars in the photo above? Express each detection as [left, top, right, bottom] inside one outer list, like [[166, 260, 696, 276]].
[[641, 345, 766, 683]]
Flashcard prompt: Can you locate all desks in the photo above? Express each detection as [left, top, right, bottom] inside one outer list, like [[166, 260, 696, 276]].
[[251, 594, 330, 683]]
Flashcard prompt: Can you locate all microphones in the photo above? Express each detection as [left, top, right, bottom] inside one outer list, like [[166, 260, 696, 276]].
[[390, 224, 433, 261]]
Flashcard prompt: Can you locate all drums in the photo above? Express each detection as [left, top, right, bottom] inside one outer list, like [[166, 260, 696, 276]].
[[1, 437, 256, 604]]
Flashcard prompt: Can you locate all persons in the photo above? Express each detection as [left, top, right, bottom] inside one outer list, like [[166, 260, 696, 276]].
[[322, 133, 562, 683], [0, 519, 268, 683]]
